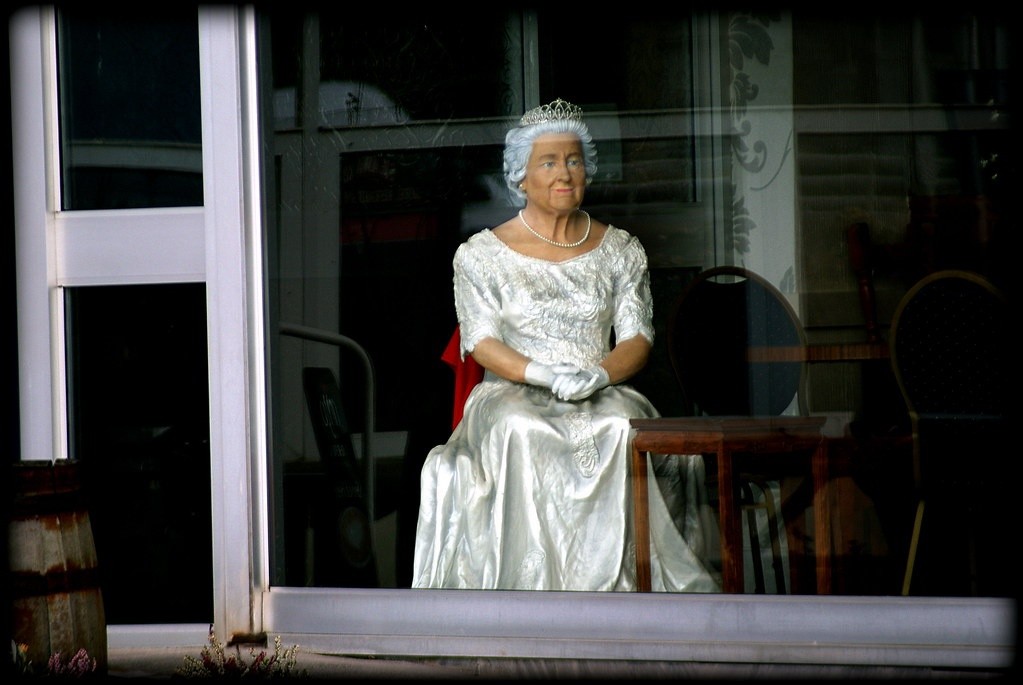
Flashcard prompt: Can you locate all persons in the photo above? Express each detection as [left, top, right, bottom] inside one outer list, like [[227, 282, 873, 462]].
[[413, 98, 724, 592]]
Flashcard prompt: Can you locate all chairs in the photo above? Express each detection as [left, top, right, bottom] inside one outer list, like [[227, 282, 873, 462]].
[[889, 266, 1023, 600], [671, 266, 815, 594]]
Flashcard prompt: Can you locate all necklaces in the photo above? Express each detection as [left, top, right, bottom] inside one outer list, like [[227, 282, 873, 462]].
[[519, 209, 590, 248]]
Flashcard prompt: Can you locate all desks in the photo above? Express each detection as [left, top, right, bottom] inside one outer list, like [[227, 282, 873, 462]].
[[632, 415, 831, 596]]
